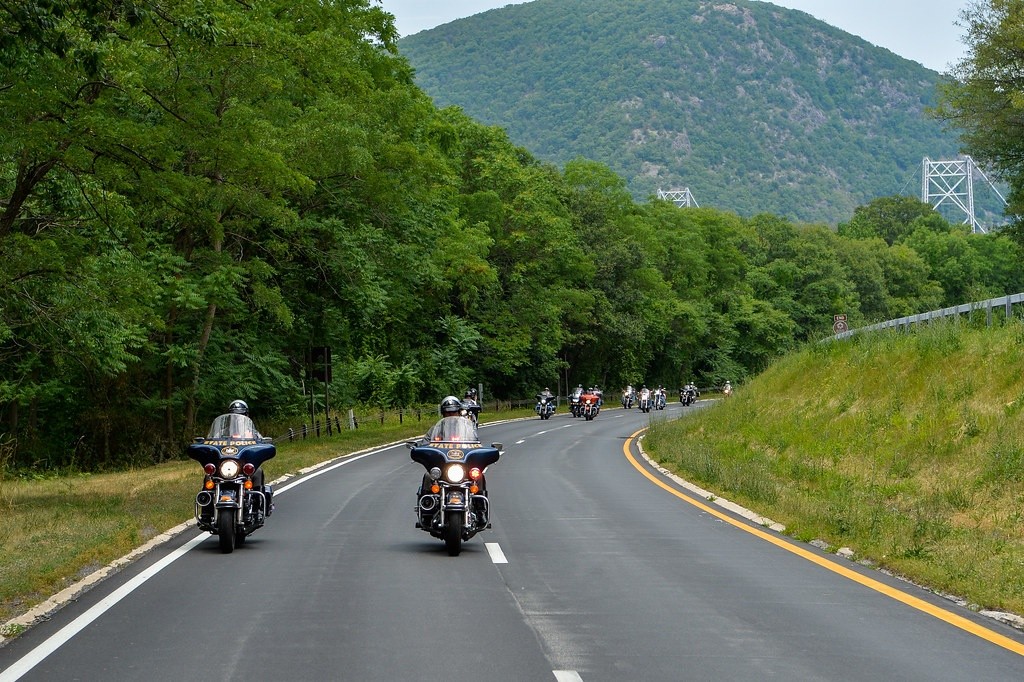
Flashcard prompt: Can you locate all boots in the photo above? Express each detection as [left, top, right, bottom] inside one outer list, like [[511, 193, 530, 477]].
[[415, 491, 436, 528], [197, 489, 213, 526], [474, 490, 491, 529], [254, 486, 265, 524]]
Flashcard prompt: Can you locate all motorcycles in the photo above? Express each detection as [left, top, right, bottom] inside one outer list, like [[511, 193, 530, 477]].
[[403, 416, 505, 557], [188, 414, 276, 554], [680, 385, 700, 406], [460, 399, 481, 427], [621, 386, 666, 416], [724, 383, 732, 398], [536, 391, 556, 419], [568, 389, 604, 421]]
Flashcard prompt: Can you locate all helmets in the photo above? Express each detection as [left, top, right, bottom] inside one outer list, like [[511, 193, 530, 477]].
[[594, 385, 598, 388], [726, 381, 730, 383], [643, 385, 646, 388], [545, 387, 549, 391], [690, 381, 694, 385], [686, 382, 689, 385], [588, 388, 593, 393], [629, 383, 632, 386], [578, 384, 582, 388], [470, 388, 477, 395], [441, 400, 462, 417], [441, 395, 462, 406], [465, 391, 472, 398], [658, 385, 661, 388], [229, 399, 249, 416]]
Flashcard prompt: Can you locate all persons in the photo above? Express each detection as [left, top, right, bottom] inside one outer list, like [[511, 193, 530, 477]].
[[535, 387, 555, 414], [569, 384, 604, 415], [621, 383, 667, 406], [197, 399, 271, 527], [722, 381, 733, 394], [680, 381, 697, 397], [406, 388, 491, 529]]
